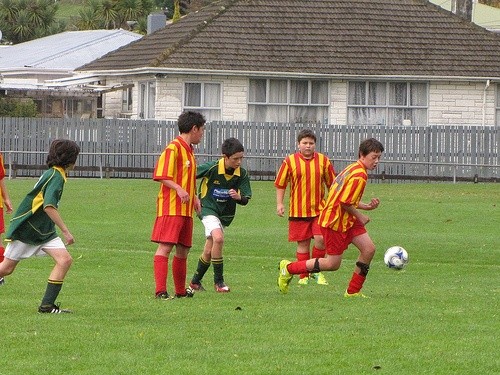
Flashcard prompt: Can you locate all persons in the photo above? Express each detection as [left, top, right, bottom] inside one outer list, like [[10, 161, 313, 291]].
[[274, 128, 386, 299], [188, 138, 252, 293], [149, 111, 207, 300], [0, 139, 80, 315]]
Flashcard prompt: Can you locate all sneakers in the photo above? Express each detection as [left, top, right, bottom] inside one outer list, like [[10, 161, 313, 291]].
[[215, 282, 230, 293], [155, 292, 174, 300], [37, 302, 73, 315], [277, 260, 293, 295], [0, 277, 4, 286], [298, 272, 329, 285], [189, 281, 206, 292], [344, 288, 369, 299], [174, 288, 194, 298]]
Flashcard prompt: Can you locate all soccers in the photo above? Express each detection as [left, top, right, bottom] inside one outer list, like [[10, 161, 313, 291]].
[[384, 246, 408, 270]]
